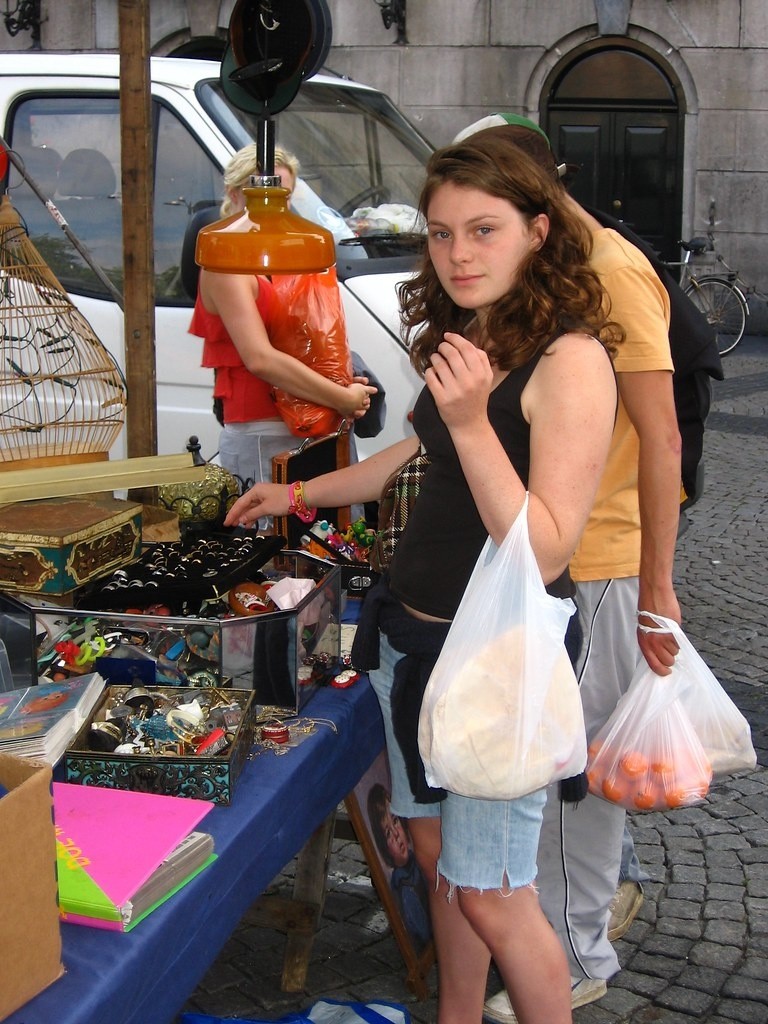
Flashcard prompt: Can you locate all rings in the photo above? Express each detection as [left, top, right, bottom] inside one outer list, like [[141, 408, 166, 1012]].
[[101, 534, 264, 592]]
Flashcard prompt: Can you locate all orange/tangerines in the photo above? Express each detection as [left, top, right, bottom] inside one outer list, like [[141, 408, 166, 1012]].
[[582, 742, 713, 811]]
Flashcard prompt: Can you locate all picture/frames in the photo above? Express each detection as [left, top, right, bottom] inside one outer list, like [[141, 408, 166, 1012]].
[[343, 750, 433, 1002]]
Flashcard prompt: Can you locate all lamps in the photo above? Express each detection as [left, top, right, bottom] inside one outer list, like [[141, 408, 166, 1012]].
[[0, 0, 50, 49], [375, 0, 407, 44]]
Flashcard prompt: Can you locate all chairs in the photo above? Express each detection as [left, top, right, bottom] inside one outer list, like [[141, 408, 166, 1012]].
[[9, 149, 60, 223], [59, 149, 120, 225]]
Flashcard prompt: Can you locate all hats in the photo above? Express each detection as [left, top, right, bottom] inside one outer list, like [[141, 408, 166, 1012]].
[[451, 111, 552, 150]]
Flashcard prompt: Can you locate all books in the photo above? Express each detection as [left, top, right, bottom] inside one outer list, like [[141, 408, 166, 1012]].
[[0, 672, 109, 772], [51, 782, 217, 934]]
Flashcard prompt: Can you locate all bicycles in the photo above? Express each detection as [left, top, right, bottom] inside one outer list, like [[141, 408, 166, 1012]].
[[624, 223, 751, 359]]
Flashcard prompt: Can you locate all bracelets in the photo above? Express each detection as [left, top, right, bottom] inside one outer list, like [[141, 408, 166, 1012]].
[[166, 708, 205, 742], [288, 478, 317, 522]]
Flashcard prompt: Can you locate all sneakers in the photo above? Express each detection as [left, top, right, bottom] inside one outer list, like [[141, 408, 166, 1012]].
[[606, 878, 644, 941], [484, 973, 608, 1023]]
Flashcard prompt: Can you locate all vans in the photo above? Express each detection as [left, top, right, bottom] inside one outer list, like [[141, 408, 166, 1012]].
[[0, 51, 434, 504]]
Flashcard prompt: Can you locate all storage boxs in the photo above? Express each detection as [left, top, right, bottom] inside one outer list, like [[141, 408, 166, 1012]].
[[272, 419, 367, 598], [63, 685, 256, 805], [0, 754, 66, 1024], [0, 497, 143, 593]]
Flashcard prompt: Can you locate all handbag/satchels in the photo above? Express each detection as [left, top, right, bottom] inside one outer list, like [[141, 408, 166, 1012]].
[[586, 610, 757, 813], [415, 492, 588, 802], [265, 266, 352, 437]]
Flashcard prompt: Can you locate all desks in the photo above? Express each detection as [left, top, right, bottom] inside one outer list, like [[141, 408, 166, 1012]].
[[0, 568, 385, 1024]]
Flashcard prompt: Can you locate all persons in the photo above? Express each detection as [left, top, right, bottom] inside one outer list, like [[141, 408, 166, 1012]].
[[188, 140, 378, 529], [449, 111, 725, 1024], [225, 133, 626, 1024]]
